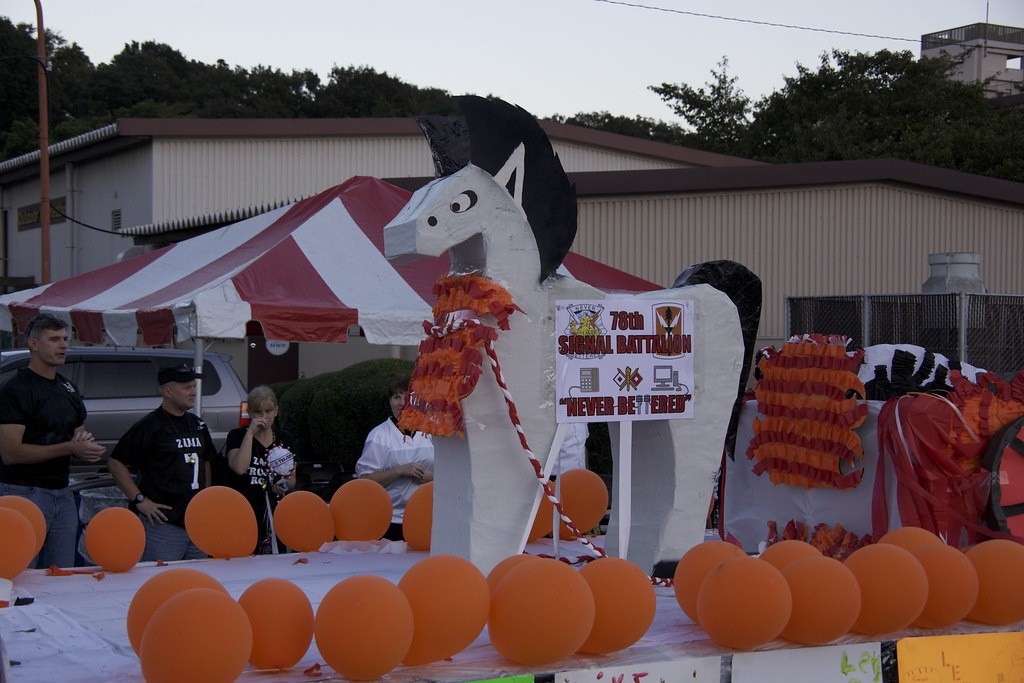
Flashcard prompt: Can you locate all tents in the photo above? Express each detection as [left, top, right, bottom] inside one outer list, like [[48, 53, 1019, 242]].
[[0, 175, 451, 419]]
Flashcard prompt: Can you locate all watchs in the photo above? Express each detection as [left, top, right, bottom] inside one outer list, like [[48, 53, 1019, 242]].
[[130, 493, 144, 504]]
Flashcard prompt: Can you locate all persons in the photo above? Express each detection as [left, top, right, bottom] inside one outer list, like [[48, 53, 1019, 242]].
[[0, 314, 108, 568], [108, 363, 216, 562], [353, 371, 433, 541], [227, 386, 295, 556]]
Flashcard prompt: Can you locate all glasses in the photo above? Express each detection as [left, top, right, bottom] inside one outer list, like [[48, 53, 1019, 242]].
[[28, 314, 57, 336]]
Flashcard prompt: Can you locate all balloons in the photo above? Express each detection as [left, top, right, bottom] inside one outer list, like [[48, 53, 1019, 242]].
[[126, 568, 314, 683], [273, 490, 335, 553], [329, 479, 393, 542], [86, 507, 146, 572], [673, 526, 1024, 650], [315, 554, 656, 681], [183, 485, 258, 558], [553, 468, 609, 535], [0, 495, 47, 580], [528, 479, 563, 543], [403, 481, 433, 551]]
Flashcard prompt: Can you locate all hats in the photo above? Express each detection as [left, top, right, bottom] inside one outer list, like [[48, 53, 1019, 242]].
[[158, 361, 205, 386]]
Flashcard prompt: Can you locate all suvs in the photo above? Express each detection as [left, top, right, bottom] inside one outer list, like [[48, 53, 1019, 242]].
[[0, 341, 250, 514]]
